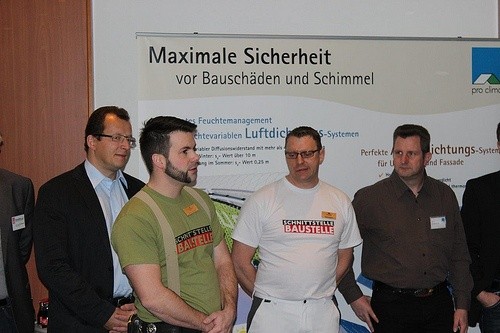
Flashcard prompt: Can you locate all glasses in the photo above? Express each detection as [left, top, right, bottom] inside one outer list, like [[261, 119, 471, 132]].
[[285, 149, 318, 159], [393, 151, 420, 158], [96, 133, 136, 148]]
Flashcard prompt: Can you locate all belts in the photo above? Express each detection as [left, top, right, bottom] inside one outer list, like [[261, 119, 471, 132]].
[[157, 324, 202, 333], [388, 285, 446, 298], [112, 296, 135, 305]]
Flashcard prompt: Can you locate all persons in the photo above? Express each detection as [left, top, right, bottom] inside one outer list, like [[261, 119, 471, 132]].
[[231, 127, 362, 333], [35, 106, 146, 333], [460, 122, 500, 333], [0, 133, 35, 333], [337, 124, 472, 333], [111, 116, 237, 333]]
